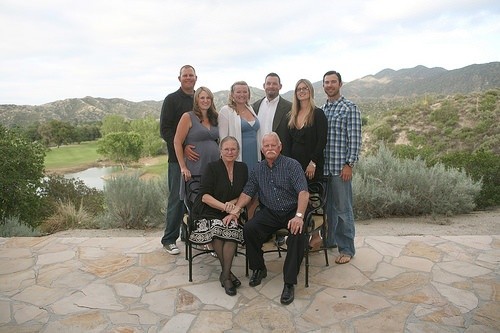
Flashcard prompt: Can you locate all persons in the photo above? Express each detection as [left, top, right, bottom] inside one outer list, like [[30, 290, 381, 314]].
[[217, 81, 261, 249], [318, 70, 363, 264], [288, 79, 328, 251], [193, 136, 248, 296], [159, 64, 199, 255], [222, 131, 309, 305], [174, 86, 220, 258], [250, 72, 293, 247]]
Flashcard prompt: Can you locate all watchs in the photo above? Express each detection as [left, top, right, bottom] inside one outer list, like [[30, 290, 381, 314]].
[[295, 212, 304, 219], [346, 162, 354, 169]]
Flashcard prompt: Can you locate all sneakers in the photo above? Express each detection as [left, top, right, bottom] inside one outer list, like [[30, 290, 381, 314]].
[[162, 243, 180, 255]]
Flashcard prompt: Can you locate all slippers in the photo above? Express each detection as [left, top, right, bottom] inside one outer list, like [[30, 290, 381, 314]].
[[336, 253, 352, 264]]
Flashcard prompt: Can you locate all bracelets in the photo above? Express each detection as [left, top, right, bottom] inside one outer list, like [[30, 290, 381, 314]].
[[309, 160, 317, 168]]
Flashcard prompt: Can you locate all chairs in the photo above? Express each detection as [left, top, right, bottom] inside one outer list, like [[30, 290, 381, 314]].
[[182, 174, 249, 282], [254, 173, 332, 288]]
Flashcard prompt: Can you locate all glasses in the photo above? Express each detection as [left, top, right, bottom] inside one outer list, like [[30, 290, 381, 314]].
[[296, 86, 309, 91]]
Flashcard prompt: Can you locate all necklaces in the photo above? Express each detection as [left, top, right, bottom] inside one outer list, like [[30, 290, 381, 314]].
[[236, 108, 248, 113]]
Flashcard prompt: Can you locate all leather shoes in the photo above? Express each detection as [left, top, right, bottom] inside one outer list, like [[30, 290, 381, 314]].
[[249, 266, 267, 286], [280, 284, 295, 304]]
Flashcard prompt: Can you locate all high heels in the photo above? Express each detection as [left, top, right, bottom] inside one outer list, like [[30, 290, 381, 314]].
[[306, 237, 323, 253], [221, 270, 241, 287], [204, 244, 217, 258], [219, 274, 237, 295]]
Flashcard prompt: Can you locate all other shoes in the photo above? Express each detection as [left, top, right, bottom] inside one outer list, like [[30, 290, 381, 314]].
[[274, 235, 286, 245]]
